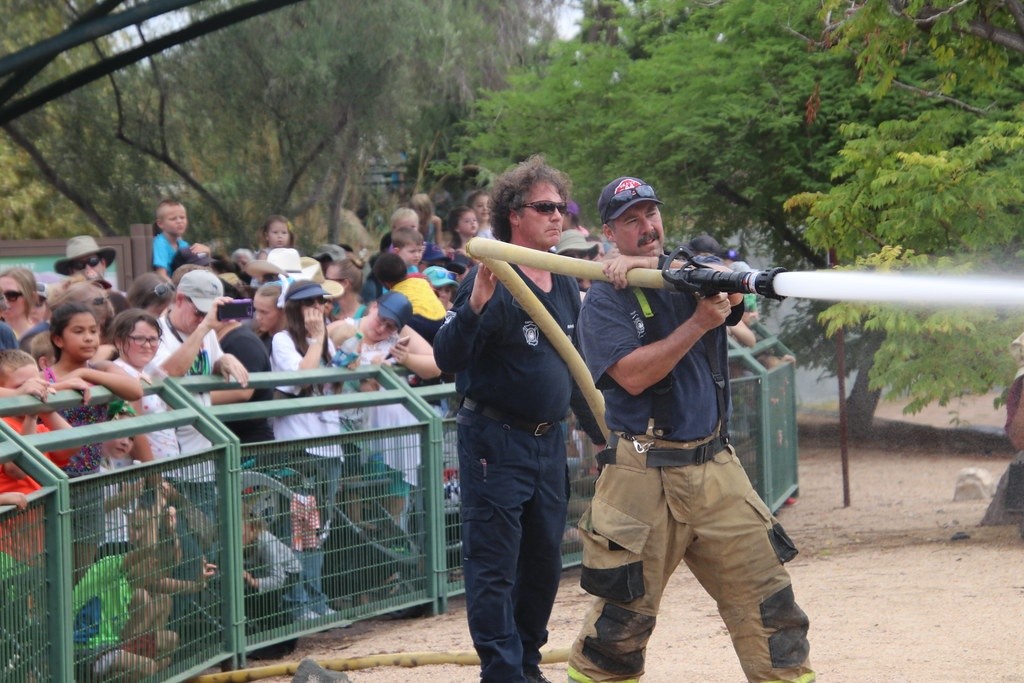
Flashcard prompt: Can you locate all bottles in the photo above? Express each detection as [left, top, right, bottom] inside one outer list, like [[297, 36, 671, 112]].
[[327, 333, 362, 367]]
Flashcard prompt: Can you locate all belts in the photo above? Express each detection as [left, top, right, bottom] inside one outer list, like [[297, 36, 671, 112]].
[[594, 430, 728, 468], [464, 398, 554, 437]]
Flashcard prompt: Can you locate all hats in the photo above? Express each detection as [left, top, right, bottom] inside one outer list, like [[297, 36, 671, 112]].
[[37, 283, 52, 300], [422, 265, 461, 289], [300, 256, 345, 298], [598, 176, 664, 223], [376, 291, 413, 334], [54, 235, 116, 276], [554, 229, 600, 260], [177, 270, 224, 314], [171, 248, 219, 269], [285, 285, 332, 302], [245, 248, 320, 281]]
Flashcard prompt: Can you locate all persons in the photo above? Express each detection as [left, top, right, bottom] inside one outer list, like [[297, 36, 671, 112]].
[[1004, 331, 1024, 451], [568, 177, 815, 683], [433, 152, 603, 683], [0, 191, 798, 683]]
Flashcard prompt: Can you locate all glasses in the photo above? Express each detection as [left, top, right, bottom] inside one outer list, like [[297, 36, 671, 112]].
[[183, 294, 207, 317], [426, 271, 458, 281], [126, 334, 163, 348], [300, 298, 326, 306], [2, 291, 24, 303], [519, 200, 569, 214], [71, 255, 103, 271], [605, 184, 656, 223]]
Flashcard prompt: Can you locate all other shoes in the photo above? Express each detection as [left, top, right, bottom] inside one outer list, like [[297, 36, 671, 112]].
[[323, 608, 354, 628], [300, 609, 331, 633]]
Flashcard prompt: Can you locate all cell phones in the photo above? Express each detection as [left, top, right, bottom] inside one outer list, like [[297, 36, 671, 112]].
[[218, 298, 253, 321]]
